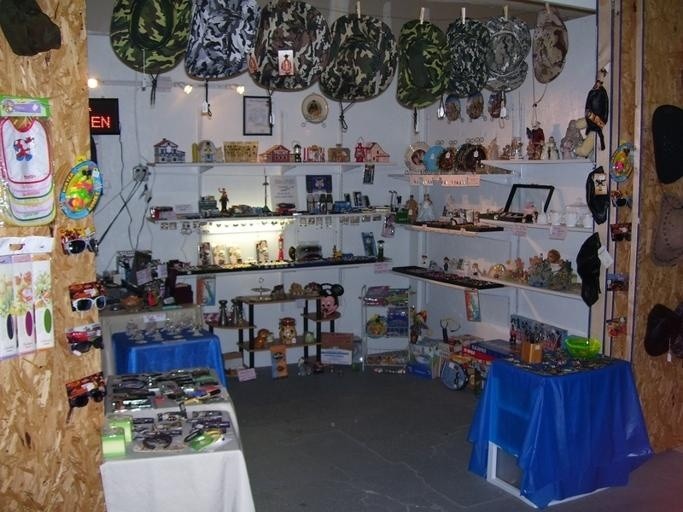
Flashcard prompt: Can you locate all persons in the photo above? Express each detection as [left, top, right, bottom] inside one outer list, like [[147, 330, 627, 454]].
[[416, 192, 436, 222], [219, 187, 231, 218], [405, 195, 419, 224]]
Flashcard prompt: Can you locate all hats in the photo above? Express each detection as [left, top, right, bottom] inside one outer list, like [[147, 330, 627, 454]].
[[644, 302, 683, 360], [319, 13, 396, 103], [586, 166, 609, 224], [59, 160, 103, 218], [577, 232, 601, 307], [185, 1, 260, 79], [485, 16, 532, 92], [609, 141, 634, 182], [653, 106, 683, 184], [398, 20, 450, 109], [249, 1, 332, 91], [652, 192, 683, 266], [111, 0, 192, 75], [448, 18, 492, 96], [533, 9, 568, 83], [586, 85, 609, 131]]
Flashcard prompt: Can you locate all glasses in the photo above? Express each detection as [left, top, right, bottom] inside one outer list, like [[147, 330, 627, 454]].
[[112, 368, 225, 410], [615, 196, 633, 208], [607, 326, 627, 335], [132, 410, 230, 449], [605, 281, 630, 292], [611, 230, 633, 240], [72, 295, 106, 312], [63, 239, 99, 255], [66, 389, 107, 408], [65, 335, 103, 353]]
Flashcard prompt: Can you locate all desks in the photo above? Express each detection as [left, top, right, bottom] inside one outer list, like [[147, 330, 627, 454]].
[[99, 305, 203, 376], [99, 410, 255, 512], [467, 348, 654, 510], [109, 325, 226, 390], [105, 368, 240, 444]]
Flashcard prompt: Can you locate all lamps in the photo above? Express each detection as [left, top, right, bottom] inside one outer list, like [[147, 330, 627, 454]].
[[179, 82, 192, 93], [230, 84, 244, 94]]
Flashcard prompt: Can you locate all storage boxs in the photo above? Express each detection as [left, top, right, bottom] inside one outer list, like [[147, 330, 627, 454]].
[[321, 331, 354, 365]]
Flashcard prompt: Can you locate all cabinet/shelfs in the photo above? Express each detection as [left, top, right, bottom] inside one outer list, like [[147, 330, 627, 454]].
[[385, 158, 596, 342], [361, 285, 411, 372], [204, 290, 341, 375], [144, 161, 393, 278]]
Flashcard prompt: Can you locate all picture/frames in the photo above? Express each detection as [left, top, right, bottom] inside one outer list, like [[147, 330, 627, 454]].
[[242, 95, 272, 136]]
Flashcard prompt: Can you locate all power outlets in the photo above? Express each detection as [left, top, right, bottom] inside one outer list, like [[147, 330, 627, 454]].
[[133, 165, 148, 182]]
